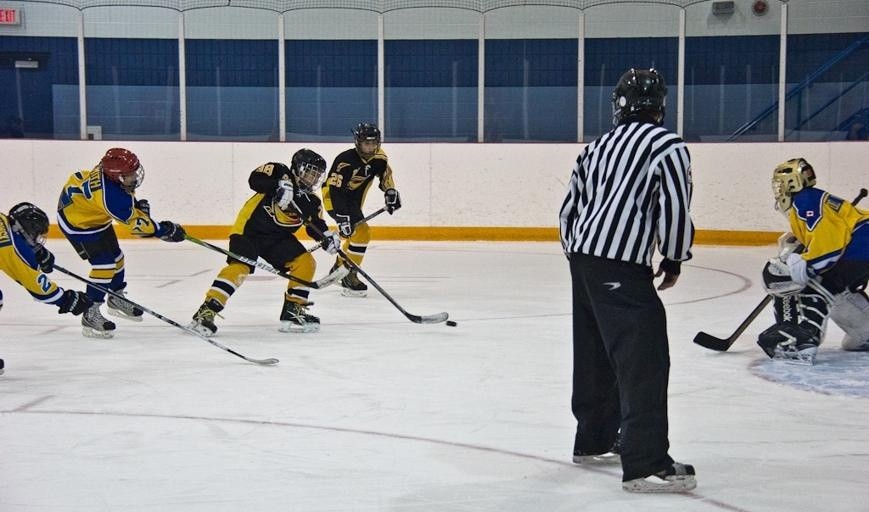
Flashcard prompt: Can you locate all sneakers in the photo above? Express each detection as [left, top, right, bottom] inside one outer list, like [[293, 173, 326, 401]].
[[786, 335, 821, 351], [645, 459, 695, 480]]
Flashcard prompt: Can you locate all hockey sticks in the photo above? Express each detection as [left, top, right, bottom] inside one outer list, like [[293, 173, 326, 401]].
[[693, 188, 869, 351], [53, 265, 279, 365], [303, 220, 449, 324], [182, 232, 351, 289]]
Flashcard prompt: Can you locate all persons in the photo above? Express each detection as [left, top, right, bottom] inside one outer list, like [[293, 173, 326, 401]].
[[193, 147, 341, 334], [757, 157, 869, 358], [0, 202, 93, 369], [559, 66, 697, 485], [56, 147, 186, 332], [321, 120, 402, 291]]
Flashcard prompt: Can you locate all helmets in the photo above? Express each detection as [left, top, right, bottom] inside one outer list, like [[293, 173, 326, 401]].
[[610, 67, 667, 128], [355, 123, 381, 159], [772, 158, 817, 212], [9, 202, 49, 245], [290, 149, 326, 195], [102, 147, 145, 190]]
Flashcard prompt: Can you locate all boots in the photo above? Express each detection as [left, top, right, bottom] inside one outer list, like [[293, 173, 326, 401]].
[[81, 300, 116, 331], [193, 297, 224, 334], [280, 291, 320, 324], [328, 256, 368, 291], [107, 283, 143, 317]]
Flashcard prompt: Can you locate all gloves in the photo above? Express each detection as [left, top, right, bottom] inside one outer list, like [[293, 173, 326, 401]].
[[153, 221, 186, 242], [34, 243, 55, 274], [276, 179, 294, 211], [57, 290, 92, 315], [134, 199, 150, 217], [336, 213, 354, 239], [385, 189, 401, 215], [321, 230, 340, 255]]
